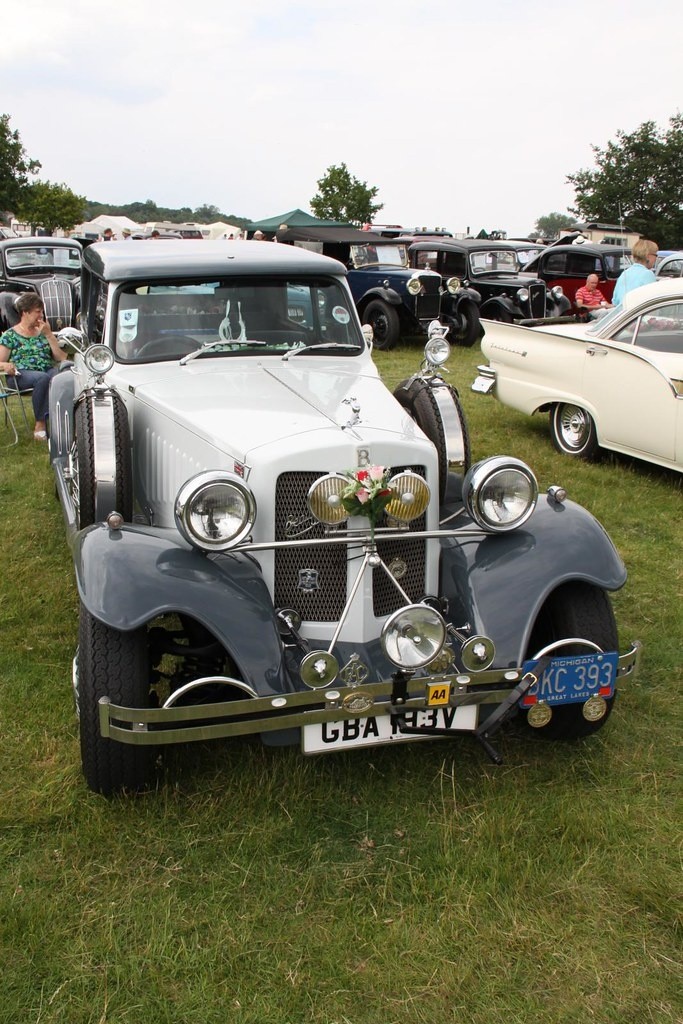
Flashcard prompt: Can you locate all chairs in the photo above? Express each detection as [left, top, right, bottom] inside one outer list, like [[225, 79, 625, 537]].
[[0, 371, 36, 450], [125, 310, 312, 360]]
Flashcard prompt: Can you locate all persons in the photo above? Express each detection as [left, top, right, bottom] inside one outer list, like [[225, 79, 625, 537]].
[[121, 227, 134, 241], [146, 230, 160, 240], [222, 233, 243, 240], [0, 292, 68, 440], [100, 228, 114, 241], [252, 230, 266, 241], [575, 273, 615, 322], [611, 239, 660, 307]]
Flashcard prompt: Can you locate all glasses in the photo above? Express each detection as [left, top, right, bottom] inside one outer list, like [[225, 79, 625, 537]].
[[648, 253, 658, 258]]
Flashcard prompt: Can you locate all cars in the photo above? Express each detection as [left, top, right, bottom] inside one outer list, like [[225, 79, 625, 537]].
[[470, 278, 682, 473], [276, 226, 683, 351], [43, 236, 643, 795], [0, 237, 85, 334]]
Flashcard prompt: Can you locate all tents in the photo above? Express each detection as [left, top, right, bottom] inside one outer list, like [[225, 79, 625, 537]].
[[245, 208, 351, 267]]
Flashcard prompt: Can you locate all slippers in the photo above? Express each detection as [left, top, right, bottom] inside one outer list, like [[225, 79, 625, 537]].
[[33, 425, 48, 443]]
